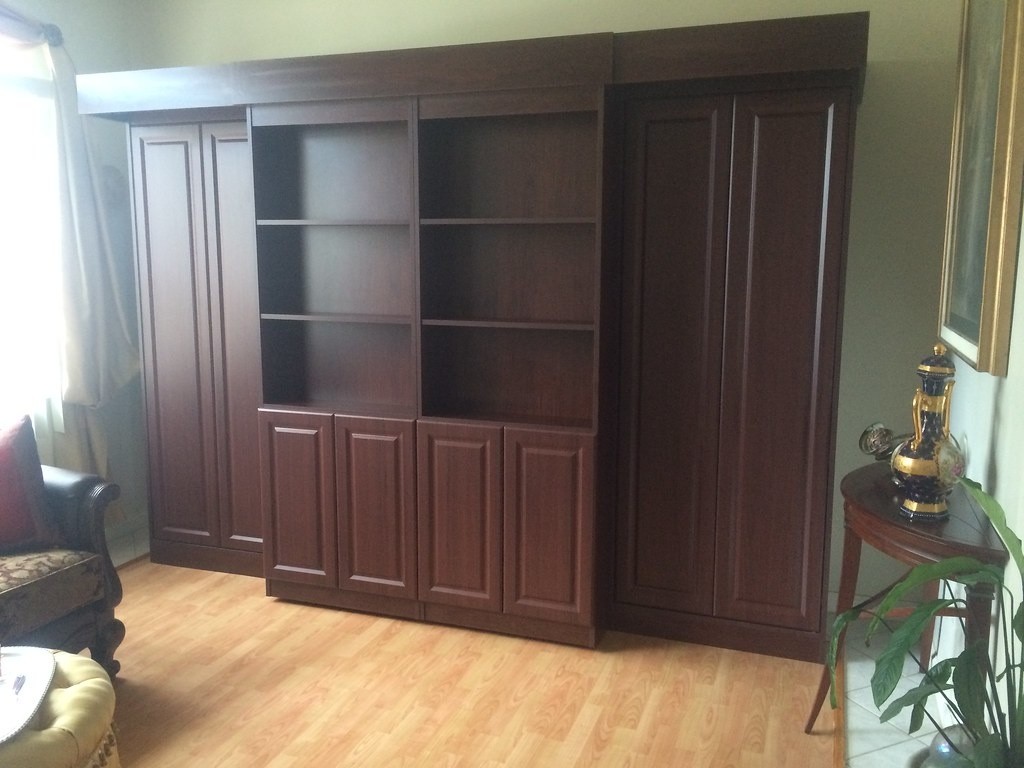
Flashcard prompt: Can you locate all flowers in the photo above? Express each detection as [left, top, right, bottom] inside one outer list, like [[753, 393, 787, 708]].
[[823, 478, 1024, 768]]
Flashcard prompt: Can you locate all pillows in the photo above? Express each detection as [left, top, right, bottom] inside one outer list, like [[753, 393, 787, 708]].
[[0, 414, 69, 553]]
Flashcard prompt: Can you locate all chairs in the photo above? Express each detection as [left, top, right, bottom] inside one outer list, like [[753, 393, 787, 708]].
[[0, 645, 123, 768]]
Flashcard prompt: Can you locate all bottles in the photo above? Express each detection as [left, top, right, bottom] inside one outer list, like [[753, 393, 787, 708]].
[[892, 343, 965, 522]]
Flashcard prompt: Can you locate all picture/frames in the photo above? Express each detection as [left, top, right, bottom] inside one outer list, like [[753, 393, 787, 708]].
[[937, 0, 1023, 380]]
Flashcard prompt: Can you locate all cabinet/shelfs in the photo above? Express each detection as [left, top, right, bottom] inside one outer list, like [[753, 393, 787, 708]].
[[251, 28, 616, 438], [257, 405, 424, 622], [415, 416, 597, 652], [602, 10, 869, 669], [75, 61, 254, 579]]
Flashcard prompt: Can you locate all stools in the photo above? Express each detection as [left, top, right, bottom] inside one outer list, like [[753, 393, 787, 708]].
[[803, 461, 1008, 736]]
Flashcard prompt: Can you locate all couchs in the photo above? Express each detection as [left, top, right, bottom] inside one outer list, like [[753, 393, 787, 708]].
[[0, 462, 126, 687]]
